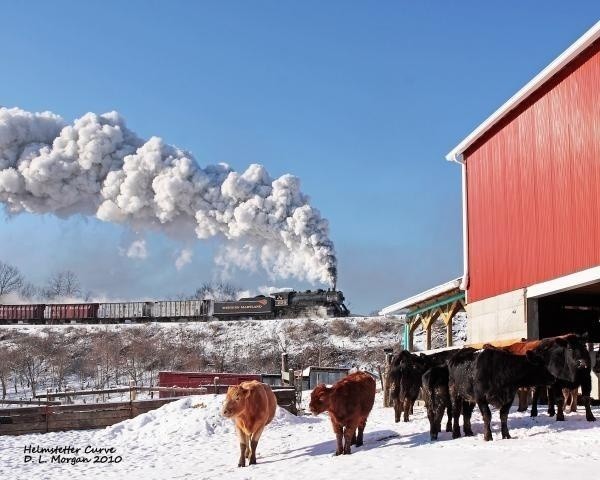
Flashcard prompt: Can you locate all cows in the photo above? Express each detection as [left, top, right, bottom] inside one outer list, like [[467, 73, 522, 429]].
[[382, 331, 597, 442], [308, 371, 377, 456], [220, 379, 278, 468]]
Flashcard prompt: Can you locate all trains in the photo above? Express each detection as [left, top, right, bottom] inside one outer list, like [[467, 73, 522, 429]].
[[1, 285, 351, 325]]
